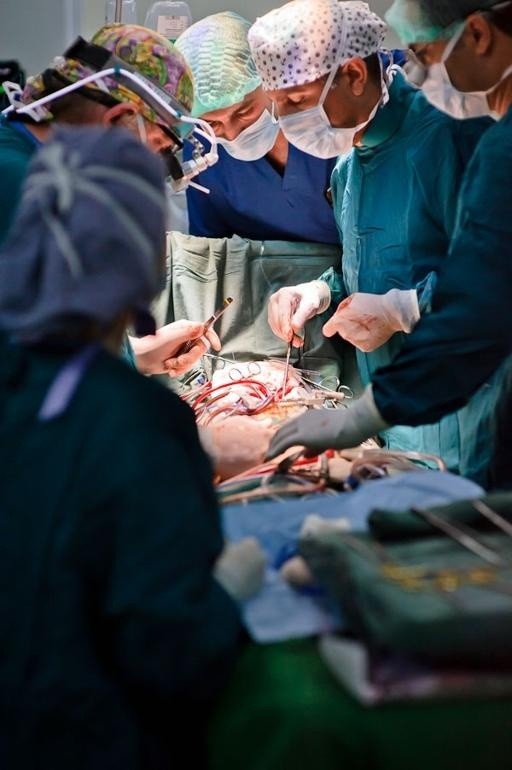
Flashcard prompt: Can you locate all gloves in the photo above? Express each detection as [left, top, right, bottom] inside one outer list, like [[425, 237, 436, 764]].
[[321, 284, 422, 354], [214, 535, 268, 608], [266, 384, 389, 461], [264, 273, 338, 349]]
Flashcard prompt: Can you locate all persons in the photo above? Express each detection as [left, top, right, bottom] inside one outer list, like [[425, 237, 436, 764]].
[[171, 4, 356, 254], [3, 121, 269, 768], [0, 21, 276, 482]]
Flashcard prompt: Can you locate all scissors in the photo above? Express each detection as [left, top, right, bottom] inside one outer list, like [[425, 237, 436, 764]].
[[202, 352, 267, 400], [318, 375, 354, 410]]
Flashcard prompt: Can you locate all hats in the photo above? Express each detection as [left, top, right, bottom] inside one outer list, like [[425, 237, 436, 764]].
[[56, 23, 195, 143], [248, 0, 387, 92], [383, 0, 495, 43], [172, 11, 262, 120]]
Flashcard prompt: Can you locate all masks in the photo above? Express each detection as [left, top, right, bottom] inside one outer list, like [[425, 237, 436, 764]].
[[421, 25, 512, 123], [271, 72, 392, 161], [208, 109, 280, 165]]
